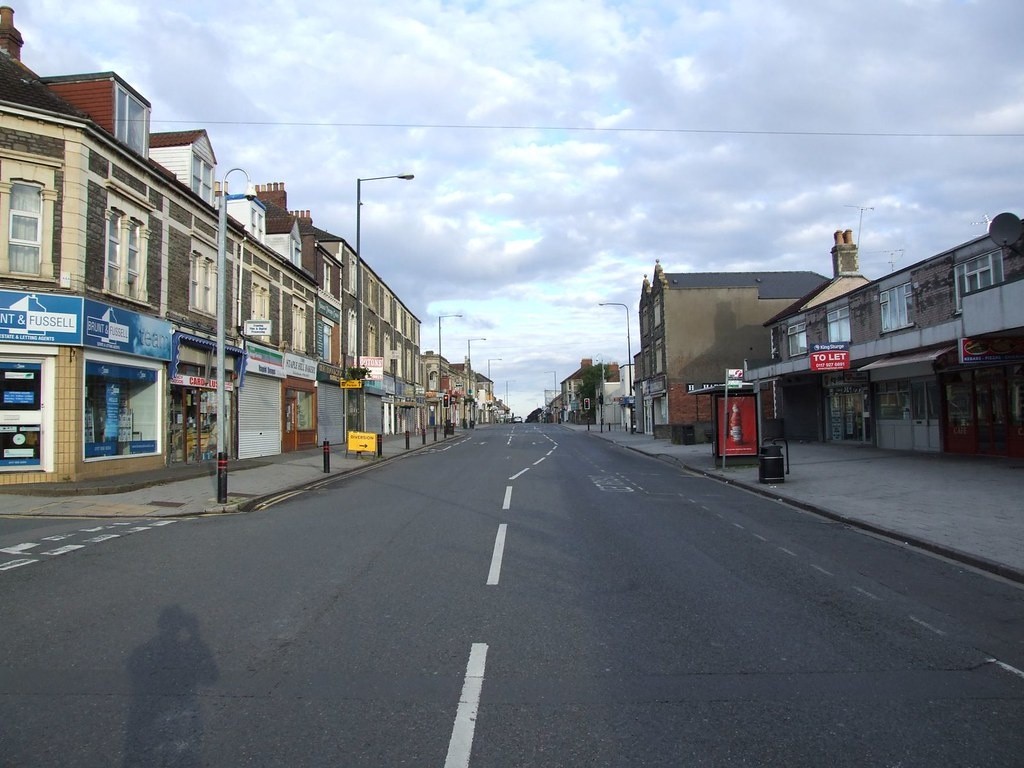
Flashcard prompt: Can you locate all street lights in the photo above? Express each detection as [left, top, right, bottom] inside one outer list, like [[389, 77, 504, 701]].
[[439, 314, 463, 429], [467, 338, 487, 429], [599, 302, 634, 436], [544, 371, 557, 407], [486, 359, 503, 400], [356, 173, 416, 459], [506, 379, 516, 408], [217, 167, 257, 504]]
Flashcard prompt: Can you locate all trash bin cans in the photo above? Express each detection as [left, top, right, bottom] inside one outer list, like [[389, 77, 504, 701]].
[[471, 421, 474, 428], [447, 423, 455, 435], [682, 425, 696, 445], [758, 445, 785, 484]]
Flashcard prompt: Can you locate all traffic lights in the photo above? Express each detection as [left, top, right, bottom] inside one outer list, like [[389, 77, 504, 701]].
[[442, 394, 450, 409], [598, 394, 604, 405], [584, 398, 590, 411]]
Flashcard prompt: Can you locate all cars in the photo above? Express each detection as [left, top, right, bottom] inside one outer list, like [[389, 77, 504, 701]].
[[513, 416, 523, 423]]
